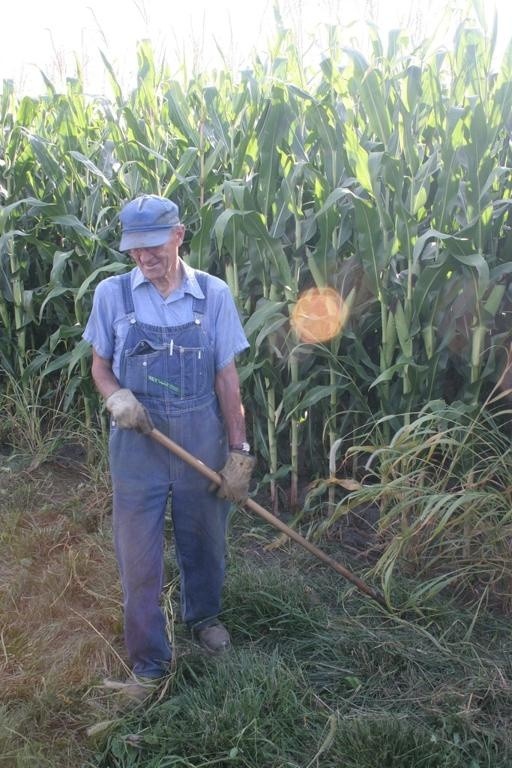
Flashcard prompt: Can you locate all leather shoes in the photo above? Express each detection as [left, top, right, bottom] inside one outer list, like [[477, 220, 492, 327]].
[[194, 618, 231, 655], [113, 673, 161, 714]]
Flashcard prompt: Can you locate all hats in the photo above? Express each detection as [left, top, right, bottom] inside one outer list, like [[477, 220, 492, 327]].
[[119, 195, 180, 253]]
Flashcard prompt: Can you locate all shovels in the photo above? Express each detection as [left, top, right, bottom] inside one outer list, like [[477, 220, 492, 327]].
[[151, 426, 389, 614]]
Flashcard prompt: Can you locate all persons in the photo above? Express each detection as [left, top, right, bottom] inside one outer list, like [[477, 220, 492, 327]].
[[82, 194, 256, 710]]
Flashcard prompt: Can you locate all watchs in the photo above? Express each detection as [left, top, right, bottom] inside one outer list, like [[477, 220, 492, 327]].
[[229, 443, 251, 452]]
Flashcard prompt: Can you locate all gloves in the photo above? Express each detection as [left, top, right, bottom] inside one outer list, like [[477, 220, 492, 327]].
[[208, 453, 256, 507], [105, 389, 153, 436]]
